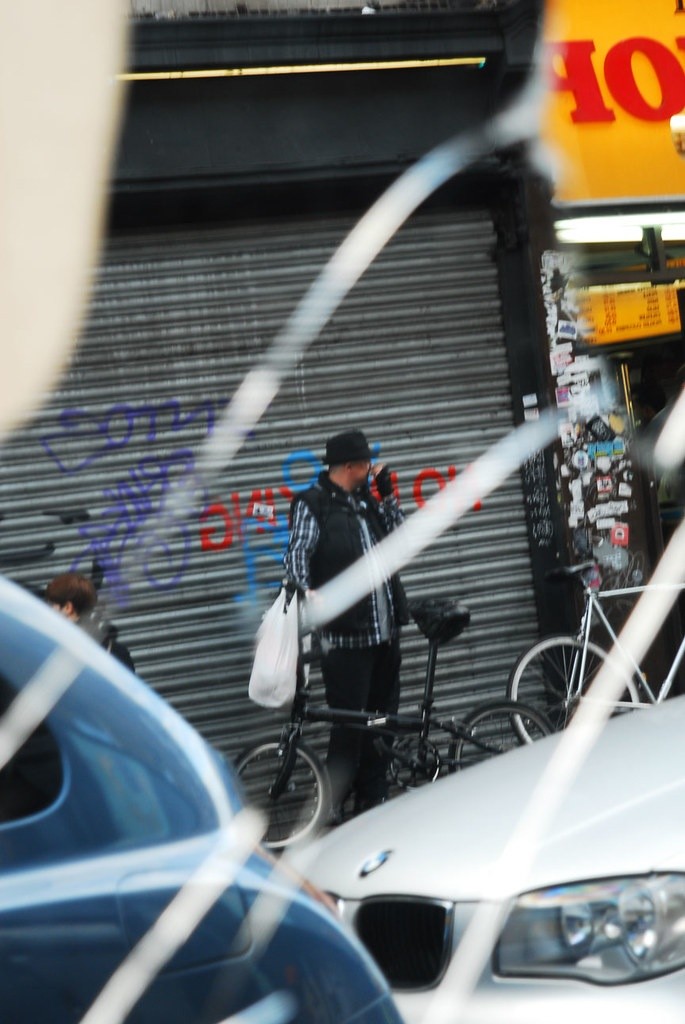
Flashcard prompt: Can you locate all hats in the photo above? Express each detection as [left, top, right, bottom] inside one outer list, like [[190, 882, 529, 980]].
[[321, 431, 378, 466]]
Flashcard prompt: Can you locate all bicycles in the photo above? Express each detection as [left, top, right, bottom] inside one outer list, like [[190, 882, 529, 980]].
[[233, 557, 685, 857]]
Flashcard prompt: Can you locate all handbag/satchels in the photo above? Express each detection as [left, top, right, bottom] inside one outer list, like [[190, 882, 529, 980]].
[[248, 587, 311, 707]]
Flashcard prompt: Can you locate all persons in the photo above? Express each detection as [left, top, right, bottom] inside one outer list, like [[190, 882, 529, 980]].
[[48, 573, 135, 672], [280, 430, 409, 827]]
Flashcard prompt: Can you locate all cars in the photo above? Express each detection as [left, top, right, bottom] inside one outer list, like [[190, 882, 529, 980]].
[[0, 573, 685, 1024]]
